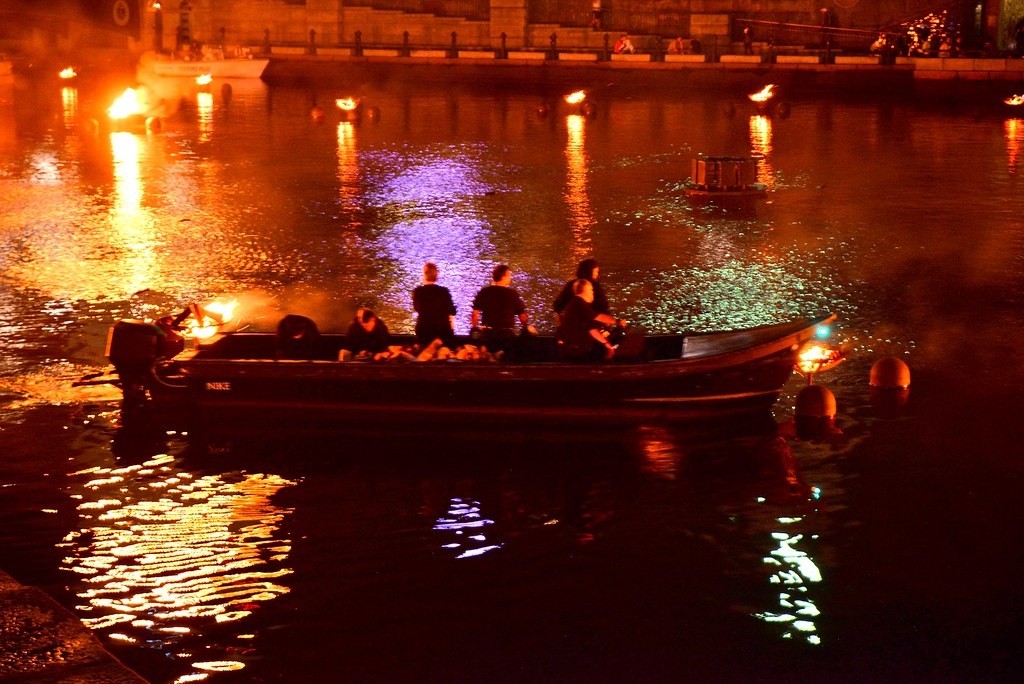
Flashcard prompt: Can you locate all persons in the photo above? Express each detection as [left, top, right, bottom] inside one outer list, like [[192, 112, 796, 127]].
[[337, 307, 392, 362], [551, 257, 611, 336], [471, 264, 539, 335], [554, 276, 627, 362], [870, 20, 953, 59], [614, 34, 702, 55], [274, 314, 322, 359], [410, 263, 457, 334]]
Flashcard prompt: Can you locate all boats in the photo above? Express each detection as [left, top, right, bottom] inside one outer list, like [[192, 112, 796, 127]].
[[151, 45, 270, 79], [72, 302, 836, 441], [684, 156, 768, 203]]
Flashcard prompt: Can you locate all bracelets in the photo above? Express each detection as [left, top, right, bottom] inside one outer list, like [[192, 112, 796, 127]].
[[616, 318, 620, 325], [603, 340, 610, 345]]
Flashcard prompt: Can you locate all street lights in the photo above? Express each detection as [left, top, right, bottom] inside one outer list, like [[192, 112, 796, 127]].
[[820, 6, 831, 63]]
